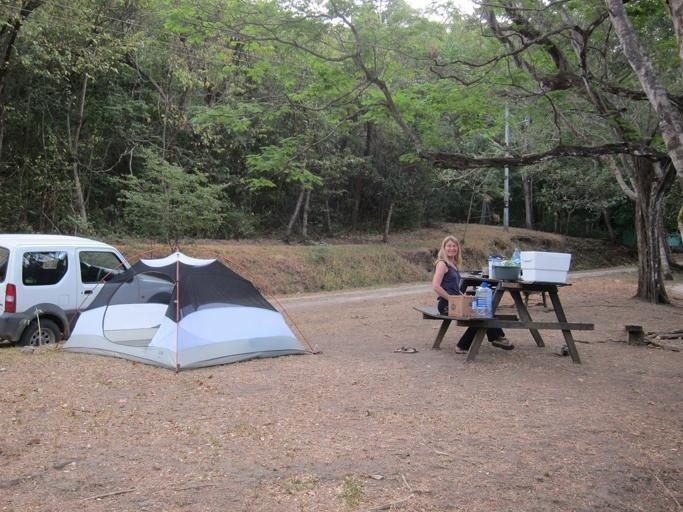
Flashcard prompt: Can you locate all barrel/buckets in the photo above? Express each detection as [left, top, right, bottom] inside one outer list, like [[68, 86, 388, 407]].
[[475, 282, 494, 318]]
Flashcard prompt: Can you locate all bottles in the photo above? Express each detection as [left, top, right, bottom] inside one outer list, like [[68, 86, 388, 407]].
[[511, 249, 520, 264]]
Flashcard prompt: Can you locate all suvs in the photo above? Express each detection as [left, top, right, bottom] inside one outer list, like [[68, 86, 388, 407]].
[[0, 232, 176, 348]]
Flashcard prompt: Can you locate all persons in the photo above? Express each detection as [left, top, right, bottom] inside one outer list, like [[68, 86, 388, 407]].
[[431, 235, 513, 355]]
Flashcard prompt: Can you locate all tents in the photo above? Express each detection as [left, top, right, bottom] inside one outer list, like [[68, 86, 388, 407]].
[[55, 243, 322, 373]]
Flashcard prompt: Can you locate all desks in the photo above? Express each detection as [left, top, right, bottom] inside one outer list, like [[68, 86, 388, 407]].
[[434, 272, 583, 364]]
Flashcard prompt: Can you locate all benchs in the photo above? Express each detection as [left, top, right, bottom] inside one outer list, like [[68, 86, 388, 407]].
[[411, 303, 595, 364]]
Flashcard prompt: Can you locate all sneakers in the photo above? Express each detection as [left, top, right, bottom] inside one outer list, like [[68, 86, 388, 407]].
[[455, 344, 468, 354], [491, 336, 514, 350]]
